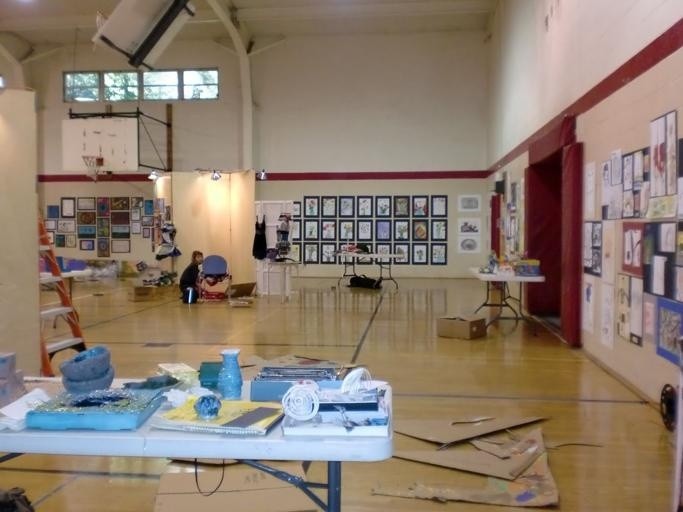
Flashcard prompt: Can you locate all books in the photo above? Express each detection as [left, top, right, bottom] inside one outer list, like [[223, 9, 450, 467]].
[[23, 388, 164, 433], [282, 401, 391, 437], [250, 366, 340, 401], [150, 394, 285, 437]]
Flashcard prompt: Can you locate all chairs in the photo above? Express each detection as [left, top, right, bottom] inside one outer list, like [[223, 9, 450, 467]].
[[198, 255, 233, 301]]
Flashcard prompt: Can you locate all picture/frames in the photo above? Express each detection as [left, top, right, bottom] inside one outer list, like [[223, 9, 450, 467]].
[[339, 220, 355, 241], [430, 219, 448, 241], [339, 243, 356, 264], [97, 218, 110, 237], [303, 195, 320, 218], [157, 198, 164, 213], [65, 234, 77, 248], [303, 241, 319, 264], [431, 243, 447, 265], [304, 219, 319, 240], [285, 242, 302, 265], [131, 197, 143, 208], [130, 207, 141, 221], [111, 211, 130, 224], [393, 196, 410, 218], [144, 200, 154, 216], [143, 228, 151, 238], [293, 201, 301, 218], [375, 196, 391, 218], [142, 216, 154, 226], [375, 242, 391, 265], [412, 219, 428, 241], [412, 195, 429, 217], [77, 225, 96, 239], [43, 219, 56, 230], [357, 243, 373, 263], [80, 240, 94, 249], [60, 197, 75, 217], [430, 195, 448, 218], [411, 243, 428, 265], [356, 196, 373, 217], [321, 219, 336, 241], [110, 197, 128, 209], [131, 222, 141, 234], [111, 225, 131, 238], [375, 220, 392, 241], [46, 231, 54, 244], [77, 197, 95, 210], [394, 219, 410, 241], [111, 240, 131, 253], [77, 212, 95, 224], [290, 219, 301, 241], [97, 197, 109, 217], [339, 196, 355, 218], [47, 205, 59, 218], [57, 220, 76, 233], [55, 234, 65, 248], [394, 243, 410, 264], [321, 242, 336, 264], [97, 238, 110, 258], [321, 195, 337, 218], [357, 220, 373, 241], [163, 205, 172, 221]]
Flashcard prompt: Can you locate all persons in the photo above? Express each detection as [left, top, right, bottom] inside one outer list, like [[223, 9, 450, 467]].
[[179, 249, 203, 305]]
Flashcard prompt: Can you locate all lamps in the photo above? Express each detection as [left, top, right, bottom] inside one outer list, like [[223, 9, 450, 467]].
[[211, 171, 221, 180], [257, 169, 268, 181], [146, 170, 159, 180]]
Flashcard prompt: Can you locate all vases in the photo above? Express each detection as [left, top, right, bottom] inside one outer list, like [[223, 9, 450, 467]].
[[218, 348, 243, 401]]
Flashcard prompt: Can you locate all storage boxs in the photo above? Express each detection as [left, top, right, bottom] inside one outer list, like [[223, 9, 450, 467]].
[[436, 314, 487, 340], [128, 285, 156, 302]]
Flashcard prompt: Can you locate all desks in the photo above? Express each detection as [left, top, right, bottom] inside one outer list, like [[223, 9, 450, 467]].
[[1, 375, 396, 512], [325, 251, 404, 290], [470, 265, 545, 339], [266, 262, 303, 304], [40, 267, 95, 328]]
